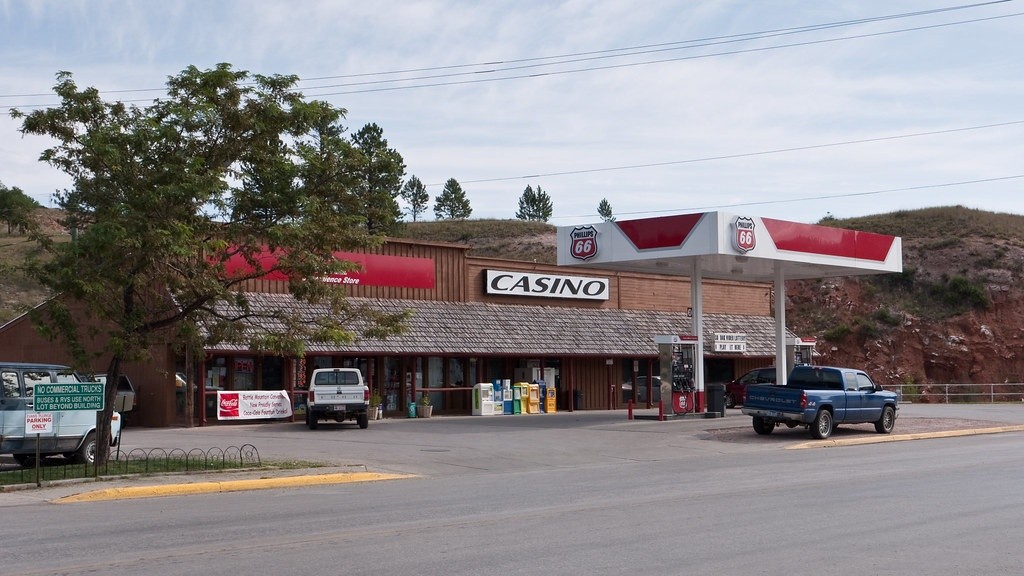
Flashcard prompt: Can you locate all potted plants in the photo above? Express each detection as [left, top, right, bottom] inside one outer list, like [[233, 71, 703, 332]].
[[416, 391, 433, 417], [368, 389, 381, 420]]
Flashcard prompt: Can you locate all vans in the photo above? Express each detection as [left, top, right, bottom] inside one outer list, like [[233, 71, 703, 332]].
[[0, 362, 122, 469]]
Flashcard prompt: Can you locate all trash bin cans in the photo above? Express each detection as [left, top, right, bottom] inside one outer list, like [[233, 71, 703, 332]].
[[472, 379, 557, 415], [567, 389, 583, 410], [707, 383, 727, 417], [408, 402, 416, 417]]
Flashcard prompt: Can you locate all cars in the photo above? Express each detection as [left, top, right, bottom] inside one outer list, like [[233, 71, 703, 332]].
[[622, 375, 690, 404], [173, 372, 225, 413], [724, 364, 776, 409]]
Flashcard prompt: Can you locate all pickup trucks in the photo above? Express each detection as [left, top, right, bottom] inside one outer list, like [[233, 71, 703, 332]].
[[740, 363, 900, 440], [302, 368, 370, 430]]
